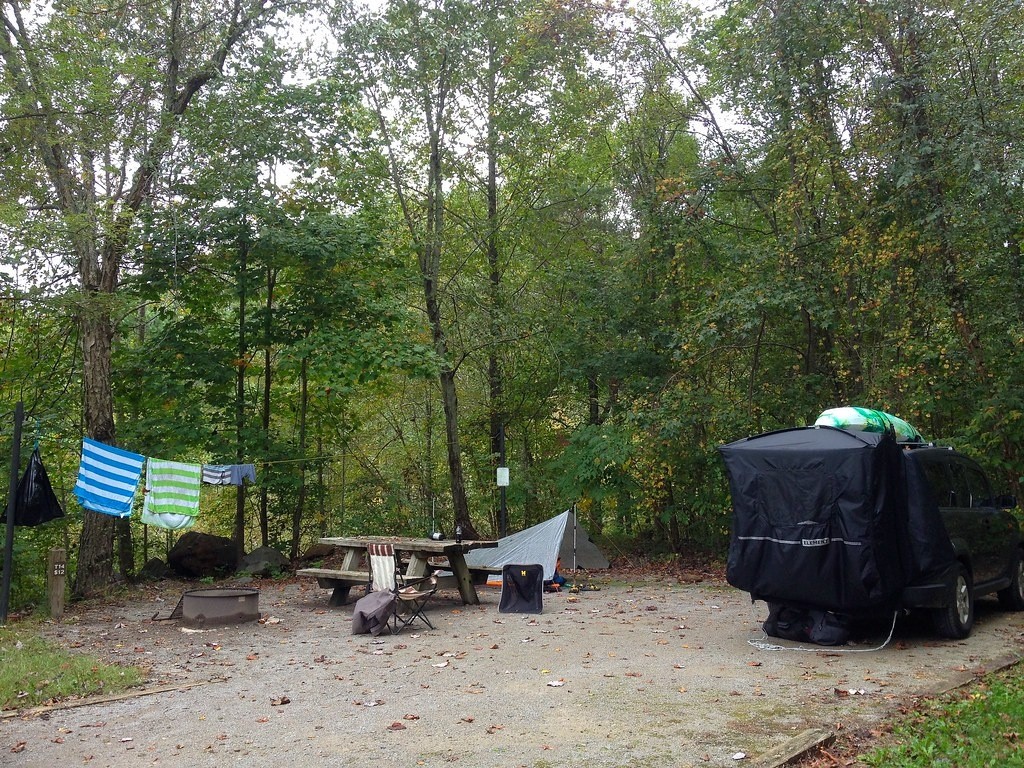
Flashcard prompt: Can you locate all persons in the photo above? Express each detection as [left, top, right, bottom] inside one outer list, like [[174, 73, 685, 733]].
[[543, 556, 568, 594]]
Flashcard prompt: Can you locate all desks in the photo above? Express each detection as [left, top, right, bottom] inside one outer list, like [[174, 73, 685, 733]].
[[317, 534, 499, 616]]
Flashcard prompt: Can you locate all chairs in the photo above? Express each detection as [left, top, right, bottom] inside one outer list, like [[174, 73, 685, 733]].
[[366, 542, 438, 635]]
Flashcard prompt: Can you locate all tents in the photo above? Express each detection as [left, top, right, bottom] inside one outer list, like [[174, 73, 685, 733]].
[[434, 500, 637, 589]]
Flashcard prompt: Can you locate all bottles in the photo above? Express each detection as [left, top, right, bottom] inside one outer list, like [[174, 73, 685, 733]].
[[429, 532, 444, 541], [455, 524, 462, 543]]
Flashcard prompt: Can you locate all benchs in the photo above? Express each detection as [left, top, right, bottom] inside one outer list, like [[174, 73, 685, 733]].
[[296, 568, 426, 616], [394, 559, 502, 605]]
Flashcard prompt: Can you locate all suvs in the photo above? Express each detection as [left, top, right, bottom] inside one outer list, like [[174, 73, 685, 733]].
[[718, 407, 1024, 648]]
